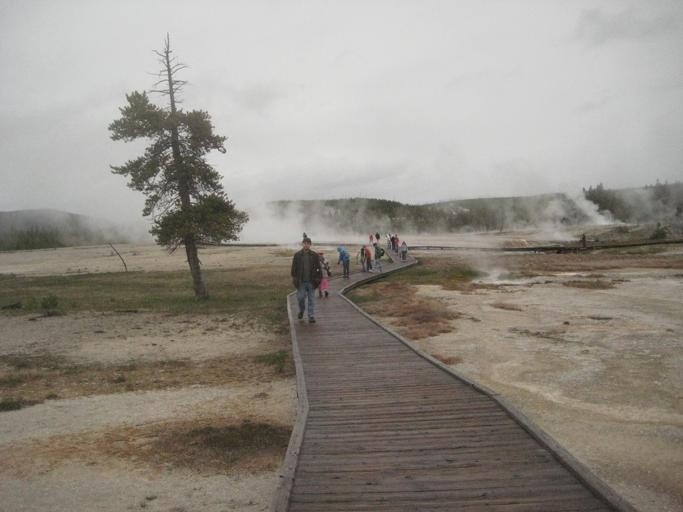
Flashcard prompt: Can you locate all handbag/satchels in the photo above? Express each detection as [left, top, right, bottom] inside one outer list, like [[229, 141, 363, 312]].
[[380, 248, 385, 256]]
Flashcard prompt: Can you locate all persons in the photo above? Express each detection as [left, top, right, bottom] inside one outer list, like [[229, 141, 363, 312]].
[[290, 237, 322, 324], [336, 245, 350, 279], [315, 252, 332, 298], [358, 231, 408, 274]]
